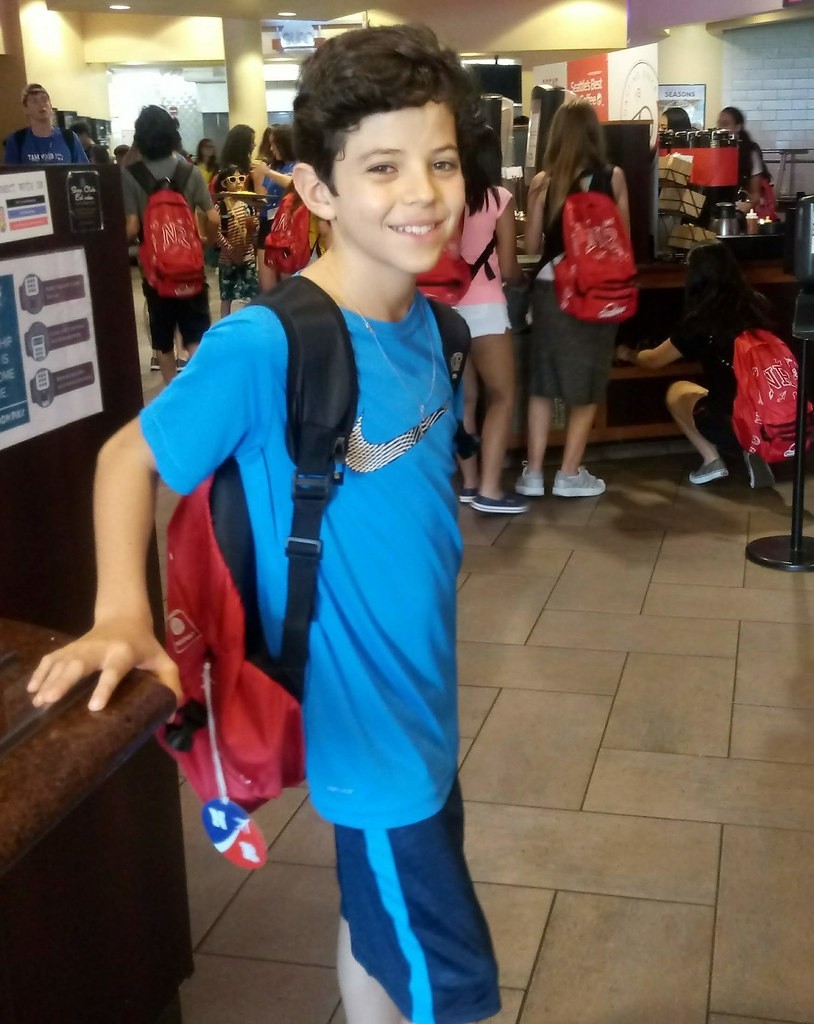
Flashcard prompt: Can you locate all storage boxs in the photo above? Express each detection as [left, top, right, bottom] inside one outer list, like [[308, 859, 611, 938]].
[[667, 224, 719, 250], [658, 154, 693, 188], [658, 187, 711, 219]]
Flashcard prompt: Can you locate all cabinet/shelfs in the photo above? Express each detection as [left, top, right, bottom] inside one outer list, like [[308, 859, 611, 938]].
[[481, 258, 804, 452]]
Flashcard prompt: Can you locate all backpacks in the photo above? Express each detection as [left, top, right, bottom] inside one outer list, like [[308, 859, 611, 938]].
[[126, 159, 204, 299], [552, 164, 639, 323], [209, 164, 238, 204], [414, 208, 497, 306], [742, 143, 778, 225], [264, 185, 321, 273], [153, 269, 471, 814], [709, 327, 814, 463]]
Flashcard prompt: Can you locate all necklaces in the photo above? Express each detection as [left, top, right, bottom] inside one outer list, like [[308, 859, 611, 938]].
[[355, 308, 435, 414]]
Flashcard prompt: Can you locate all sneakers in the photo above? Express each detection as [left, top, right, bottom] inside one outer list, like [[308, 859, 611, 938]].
[[688, 459, 729, 485], [471, 493, 529, 514], [744, 451, 774, 489], [553, 465, 606, 497], [459, 488, 478, 502], [514, 461, 544, 497]]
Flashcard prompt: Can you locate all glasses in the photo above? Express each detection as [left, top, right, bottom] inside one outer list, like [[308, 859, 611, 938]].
[[223, 175, 246, 183]]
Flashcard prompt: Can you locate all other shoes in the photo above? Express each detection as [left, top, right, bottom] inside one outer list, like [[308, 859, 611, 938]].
[[151, 357, 159, 370], [176, 359, 188, 371], [214, 267, 219, 276]]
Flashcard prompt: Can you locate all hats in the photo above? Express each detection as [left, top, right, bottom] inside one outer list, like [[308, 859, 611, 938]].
[[21, 83, 52, 107]]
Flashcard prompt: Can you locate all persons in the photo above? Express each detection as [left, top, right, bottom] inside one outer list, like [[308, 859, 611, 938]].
[[114, 145, 130, 162], [658, 108, 692, 131], [69, 122, 96, 152], [192, 140, 219, 186], [4, 83, 89, 165], [120, 105, 220, 386], [89, 144, 110, 165], [615, 240, 775, 492], [218, 125, 257, 171], [416, 128, 530, 515], [718, 107, 763, 201], [251, 124, 299, 295], [26, 23, 502, 1024], [214, 164, 260, 321], [515, 99, 630, 497]]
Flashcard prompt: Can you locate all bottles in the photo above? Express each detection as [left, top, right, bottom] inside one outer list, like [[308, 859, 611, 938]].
[[759, 215, 772, 234], [783, 192, 806, 274], [746, 209, 758, 234]]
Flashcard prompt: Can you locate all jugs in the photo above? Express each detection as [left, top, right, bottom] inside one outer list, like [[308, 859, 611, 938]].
[[709, 202, 739, 235], [658, 128, 737, 149]]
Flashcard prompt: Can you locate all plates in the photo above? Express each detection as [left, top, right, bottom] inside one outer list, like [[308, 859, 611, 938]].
[[516, 254, 542, 264], [213, 193, 279, 200]]
